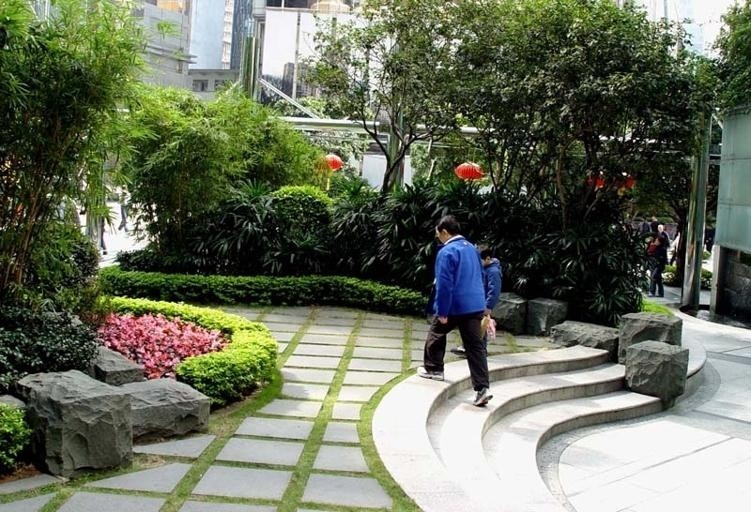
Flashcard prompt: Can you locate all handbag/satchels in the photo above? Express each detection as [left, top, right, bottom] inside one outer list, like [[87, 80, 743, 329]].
[[486, 314, 496, 340]]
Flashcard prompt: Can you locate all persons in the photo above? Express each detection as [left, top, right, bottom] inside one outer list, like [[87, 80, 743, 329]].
[[704, 224, 713, 253], [80, 197, 107, 256], [64, 197, 79, 225], [117, 189, 130, 235], [624, 212, 682, 297], [449, 248, 503, 357], [416, 214, 493, 406]]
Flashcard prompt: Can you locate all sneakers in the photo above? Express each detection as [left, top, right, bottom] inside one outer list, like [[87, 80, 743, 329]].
[[450, 346, 466, 355], [417, 367, 444, 381], [473, 387, 493, 406]]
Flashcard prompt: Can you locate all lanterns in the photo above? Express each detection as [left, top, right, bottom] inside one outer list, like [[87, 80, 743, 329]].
[[586, 173, 605, 190], [614, 173, 636, 196], [455, 161, 485, 181], [325, 150, 344, 191]]
